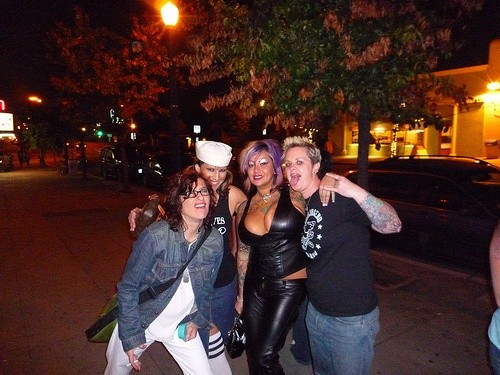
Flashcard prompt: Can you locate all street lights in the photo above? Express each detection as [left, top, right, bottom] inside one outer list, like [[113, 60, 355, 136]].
[[160, 1, 179, 157]]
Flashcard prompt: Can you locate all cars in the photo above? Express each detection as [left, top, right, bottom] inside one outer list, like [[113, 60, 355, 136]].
[[342, 169, 499, 259]]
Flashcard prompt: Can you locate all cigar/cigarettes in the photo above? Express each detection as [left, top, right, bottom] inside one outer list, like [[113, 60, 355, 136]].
[[126, 360, 138, 367]]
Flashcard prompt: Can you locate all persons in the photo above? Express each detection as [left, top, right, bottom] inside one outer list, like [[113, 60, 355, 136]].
[[234, 139, 337, 375], [128, 141, 248, 375], [280, 135, 402, 375], [487, 223, 500, 374], [104, 172, 224, 375]]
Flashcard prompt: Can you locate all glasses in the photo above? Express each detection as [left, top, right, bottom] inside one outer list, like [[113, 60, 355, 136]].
[[179, 187, 209, 198]]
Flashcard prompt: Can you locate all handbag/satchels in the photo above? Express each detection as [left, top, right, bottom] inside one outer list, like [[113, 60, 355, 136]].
[[84, 293, 118, 343], [226, 312, 247, 359], [132, 200, 168, 238]]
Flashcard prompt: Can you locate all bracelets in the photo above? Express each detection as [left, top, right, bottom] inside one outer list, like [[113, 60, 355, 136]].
[[237, 295, 243, 302]]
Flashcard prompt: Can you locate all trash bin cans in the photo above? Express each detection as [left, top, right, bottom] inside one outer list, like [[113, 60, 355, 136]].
[[68, 160, 78, 174], [485, 140, 500, 159], [59, 160, 68, 174]]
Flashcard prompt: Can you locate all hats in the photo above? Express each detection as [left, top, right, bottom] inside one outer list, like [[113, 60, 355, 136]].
[[195, 141, 233, 166]]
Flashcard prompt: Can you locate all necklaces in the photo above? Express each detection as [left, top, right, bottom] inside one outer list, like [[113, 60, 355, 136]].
[[256, 188, 273, 204]]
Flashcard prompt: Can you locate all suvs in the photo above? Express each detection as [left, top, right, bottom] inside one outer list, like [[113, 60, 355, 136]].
[[376, 154, 500, 210], [100, 147, 122, 170]]
